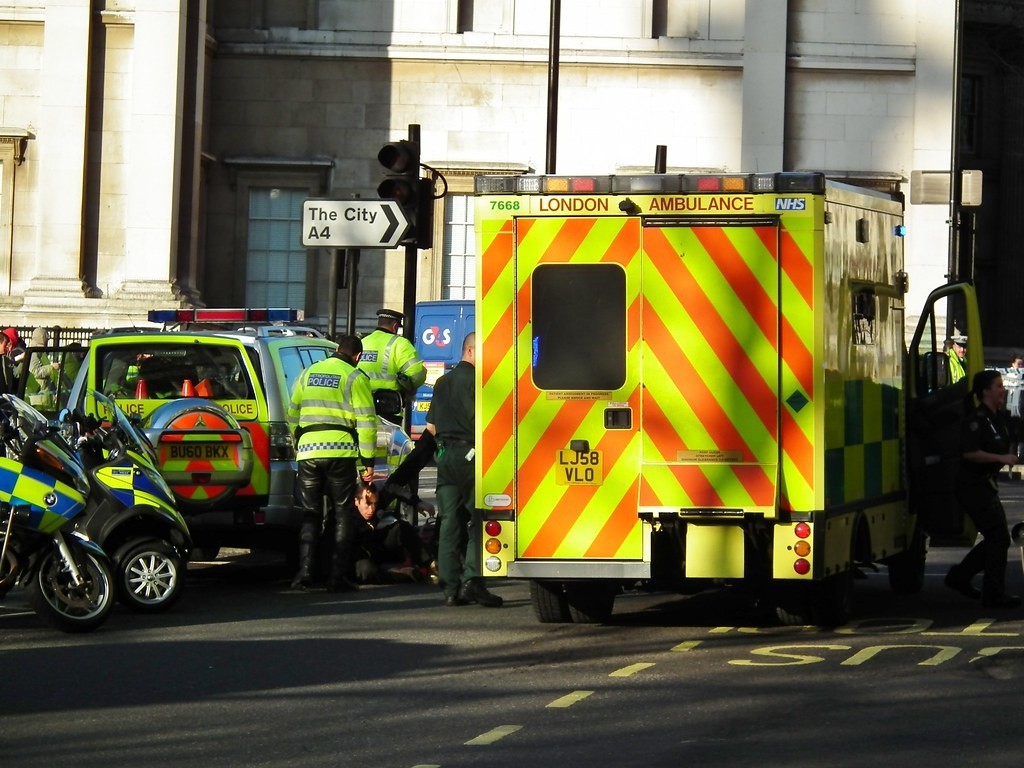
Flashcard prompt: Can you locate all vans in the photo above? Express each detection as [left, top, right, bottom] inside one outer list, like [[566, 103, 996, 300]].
[[412, 299, 474, 443]]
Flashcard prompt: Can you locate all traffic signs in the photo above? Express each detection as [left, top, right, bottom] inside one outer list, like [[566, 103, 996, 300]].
[[301, 199, 408, 248]]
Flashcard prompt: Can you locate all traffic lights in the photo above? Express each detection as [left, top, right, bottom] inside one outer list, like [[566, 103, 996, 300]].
[[377, 139, 416, 245]]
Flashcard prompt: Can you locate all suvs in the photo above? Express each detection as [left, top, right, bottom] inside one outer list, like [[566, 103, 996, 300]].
[[68, 305, 342, 564]]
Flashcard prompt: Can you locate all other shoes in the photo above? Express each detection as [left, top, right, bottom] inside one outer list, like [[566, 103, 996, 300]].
[[291, 569, 314, 590], [458, 580, 503, 608], [325, 575, 359, 592], [425, 560, 439, 586], [981, 592, 1021, 611], [445, 594, 466, 605], [946, 565, 981, 599]]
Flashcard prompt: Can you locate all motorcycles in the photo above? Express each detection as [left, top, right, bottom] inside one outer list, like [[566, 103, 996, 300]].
[[0, 388, 192, 631]]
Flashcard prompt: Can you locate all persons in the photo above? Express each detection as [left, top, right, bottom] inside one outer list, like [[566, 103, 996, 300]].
[[1003, 355, 1024, 465], [941, 371, 1024, 608], [287, 309, 503, 607], [0, 327, 224, 422], [947, 335, 968, 385]]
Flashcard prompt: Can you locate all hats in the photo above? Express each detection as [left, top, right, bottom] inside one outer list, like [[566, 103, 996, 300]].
[[377, 309, 404, 330], [951, 335, 969, 346]]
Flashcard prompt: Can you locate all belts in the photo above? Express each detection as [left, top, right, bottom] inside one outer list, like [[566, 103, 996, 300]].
[[444, 439, 470, 447]]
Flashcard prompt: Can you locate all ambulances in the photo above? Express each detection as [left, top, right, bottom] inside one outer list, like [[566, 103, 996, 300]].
[[473, 170, 988, 627]]
